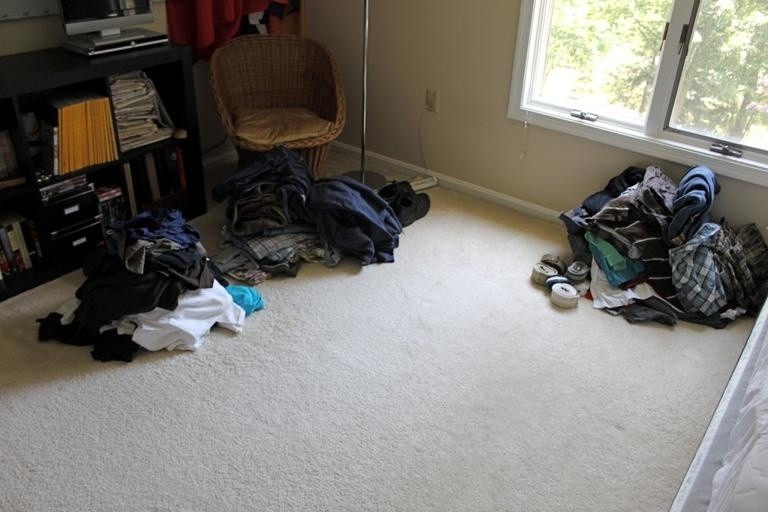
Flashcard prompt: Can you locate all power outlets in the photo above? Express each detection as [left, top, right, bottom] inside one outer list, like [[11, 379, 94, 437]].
[[421, 88, 440, 113]]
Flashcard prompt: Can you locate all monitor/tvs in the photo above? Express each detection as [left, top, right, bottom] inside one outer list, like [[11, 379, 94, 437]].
[[58, 0, 154, 47]]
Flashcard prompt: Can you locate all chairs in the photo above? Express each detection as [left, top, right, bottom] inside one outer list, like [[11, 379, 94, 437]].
[[210, 33, 348, 183]]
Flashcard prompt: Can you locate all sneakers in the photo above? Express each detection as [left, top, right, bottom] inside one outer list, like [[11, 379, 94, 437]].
[[377, 179, 415, 203], [389, 192, 431, 228]]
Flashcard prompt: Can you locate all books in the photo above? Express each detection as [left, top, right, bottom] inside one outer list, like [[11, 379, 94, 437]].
[[41, 69, 190, 222], [0, 212, 41, 283], [0, 132, 28, 190]]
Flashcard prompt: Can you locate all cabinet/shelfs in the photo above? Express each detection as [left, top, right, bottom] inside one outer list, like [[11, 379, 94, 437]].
[[0, 29, 207, 312]]
